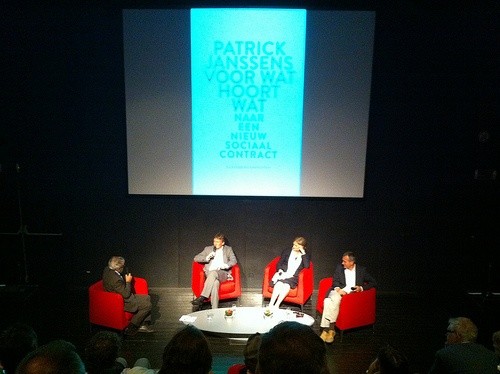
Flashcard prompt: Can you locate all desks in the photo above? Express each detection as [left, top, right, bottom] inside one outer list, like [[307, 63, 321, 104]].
[[182, 307, 315, 338]]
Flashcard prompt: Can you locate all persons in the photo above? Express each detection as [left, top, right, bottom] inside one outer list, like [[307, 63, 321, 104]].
[[492, 331, 500, 354], [429, 317, 500, 374], [0, 322, 330, 374], [365, 343, 414, 374], [190, 232, 237, 310], [102, 256, 155, 336], [264, 237, 311, 316], [319, 251, 377, 344]]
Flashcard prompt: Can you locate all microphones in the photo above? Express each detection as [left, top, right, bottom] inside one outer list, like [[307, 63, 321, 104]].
[[351, 287, 357, 290], [125, 267, 129, 276]]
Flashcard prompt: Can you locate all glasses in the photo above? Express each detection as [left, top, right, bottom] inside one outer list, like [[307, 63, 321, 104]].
[[443, 330, 455, 336]]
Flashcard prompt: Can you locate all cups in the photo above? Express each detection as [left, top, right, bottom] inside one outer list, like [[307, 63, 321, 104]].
[[207, 311, 213, 319], [232, 304, 236, 310]]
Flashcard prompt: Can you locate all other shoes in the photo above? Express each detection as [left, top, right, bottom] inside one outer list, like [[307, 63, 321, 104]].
[[123, 324, 154, 335], [190, 297, 205, 307], [320, 330, 336, 342]]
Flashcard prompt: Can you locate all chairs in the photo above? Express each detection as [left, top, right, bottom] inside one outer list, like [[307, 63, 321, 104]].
[[191, 260, 242, 303], [89, 274, 149, 330], [263, 255, 314, 312], [316, 277, 376, 343]]
